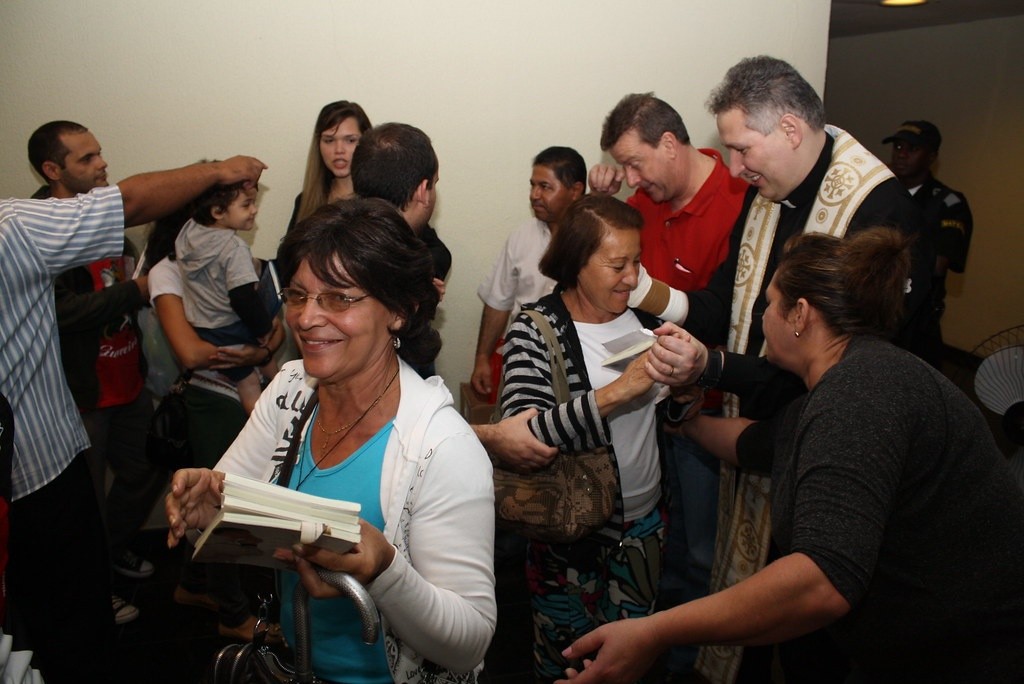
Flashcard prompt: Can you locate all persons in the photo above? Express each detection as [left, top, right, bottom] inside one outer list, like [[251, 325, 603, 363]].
[[499, 196, 703, 684], [282, 102, 451, 377], [470, 146, 591, 398], [882, 120, 973, 366], [166, 198, 496, 684], [591, 94, 749, 684], [0, 120, 267, 684], [145, 178, 286, 642], [628, 56, 913, 684], [555, 228, 1024, 684]]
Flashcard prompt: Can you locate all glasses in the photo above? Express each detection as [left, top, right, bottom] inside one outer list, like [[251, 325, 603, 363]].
[[892, 140, 921, 152], [276, 288, 371, 314]]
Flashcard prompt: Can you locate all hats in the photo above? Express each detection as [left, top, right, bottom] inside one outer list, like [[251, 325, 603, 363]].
[[881, 120, 942, 150]]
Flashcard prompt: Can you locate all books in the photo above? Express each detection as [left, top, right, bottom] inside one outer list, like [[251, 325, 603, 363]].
[[121, 242, 150, 316], [600, 335, 671, 403], [261, 260, 283, 319], [192, 473, 361, 570]]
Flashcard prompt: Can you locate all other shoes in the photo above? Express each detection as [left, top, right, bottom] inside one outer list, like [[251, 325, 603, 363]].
[[175, 585, 219, 609], [212, 616, 283, 644]]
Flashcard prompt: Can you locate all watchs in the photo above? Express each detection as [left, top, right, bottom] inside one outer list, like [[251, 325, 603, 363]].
[[698, 348, 722, 389], [257, 346, 272, 366]]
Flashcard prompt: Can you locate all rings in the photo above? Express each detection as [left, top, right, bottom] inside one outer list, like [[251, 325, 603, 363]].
[[668, 366, 676, 377]]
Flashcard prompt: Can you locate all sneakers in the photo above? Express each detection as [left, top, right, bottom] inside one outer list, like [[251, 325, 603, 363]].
[[108, 593, 140, 624], [108, 549, 153, 578]]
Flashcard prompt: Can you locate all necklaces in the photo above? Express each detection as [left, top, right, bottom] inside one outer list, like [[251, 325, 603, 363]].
[[316, 366, 398, 453]]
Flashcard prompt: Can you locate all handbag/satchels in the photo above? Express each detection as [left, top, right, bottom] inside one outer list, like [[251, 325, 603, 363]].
[[211, 620, 318, 684], [142, 388, 189, 454], [494, 309, 617, 545]]
